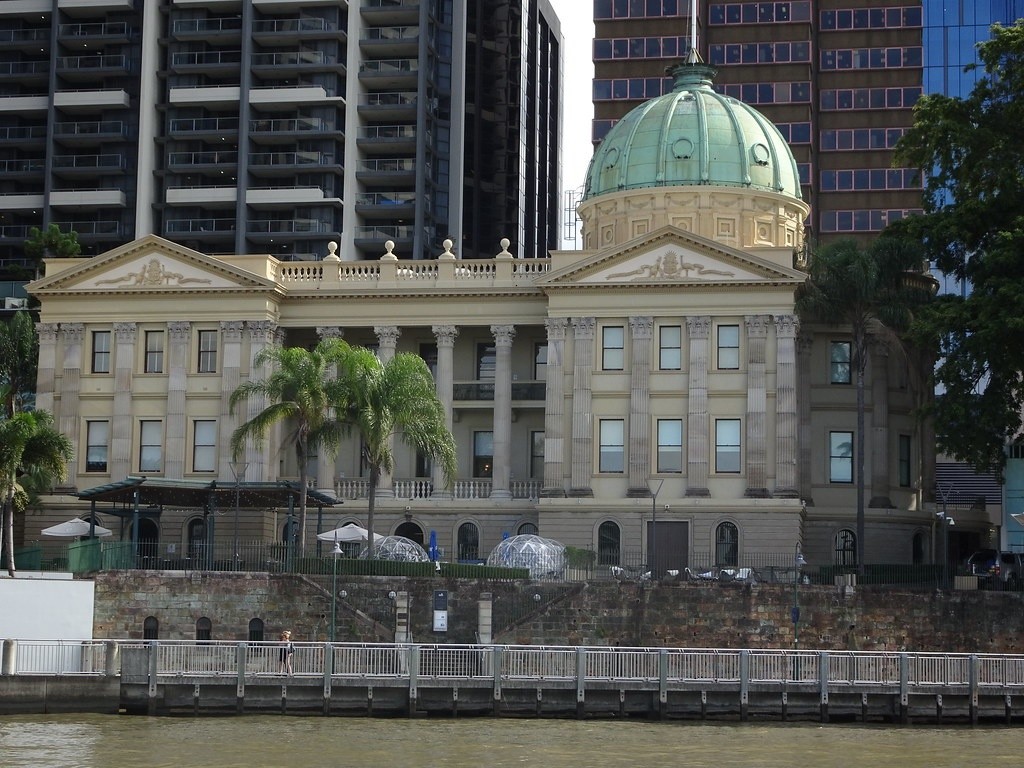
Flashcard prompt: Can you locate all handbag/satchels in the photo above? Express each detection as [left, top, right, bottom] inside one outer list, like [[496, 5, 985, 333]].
[[288, 643, 296, 653]]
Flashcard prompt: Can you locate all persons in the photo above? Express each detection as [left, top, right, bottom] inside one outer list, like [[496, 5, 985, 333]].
[[275, 631, 294, 677]]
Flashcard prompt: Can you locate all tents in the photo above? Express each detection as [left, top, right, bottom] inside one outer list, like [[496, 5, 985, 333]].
[[317, 524, 384, 545]]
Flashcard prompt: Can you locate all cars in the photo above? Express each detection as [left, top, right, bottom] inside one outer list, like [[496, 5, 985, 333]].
[[966, 549, 1023, 587]]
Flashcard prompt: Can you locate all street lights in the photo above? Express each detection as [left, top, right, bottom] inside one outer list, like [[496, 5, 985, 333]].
[[790, 539, 803, 681], [328, 531, 343, 675]]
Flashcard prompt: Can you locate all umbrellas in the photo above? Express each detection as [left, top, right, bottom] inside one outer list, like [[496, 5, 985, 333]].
[[41, 517, 112, 537], [428, 530, 438, 562], [501, 531, 509, 564]]
